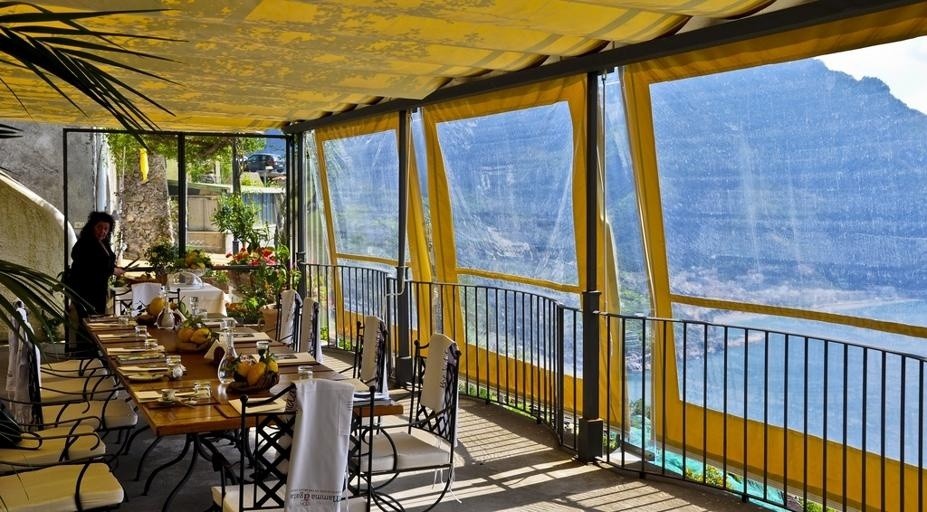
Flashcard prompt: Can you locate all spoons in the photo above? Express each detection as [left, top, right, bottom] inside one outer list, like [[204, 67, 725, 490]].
[[172, 397, 195, 409]]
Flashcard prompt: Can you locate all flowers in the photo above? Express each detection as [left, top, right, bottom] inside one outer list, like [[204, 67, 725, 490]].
[[144, 242, 213, 269], [225, 247, 278, 270]]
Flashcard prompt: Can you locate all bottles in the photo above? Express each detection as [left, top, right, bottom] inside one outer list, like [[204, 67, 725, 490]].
[[189, 296, 199, 317], [217, 330, 239, 385]]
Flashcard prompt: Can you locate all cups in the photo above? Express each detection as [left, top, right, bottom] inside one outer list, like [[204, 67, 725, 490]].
[[223, 319, 236, 329], [256, 341, 268, 350], [118, 317, 128, 325], [161, 389, 174, 401], [165, 355, 181, 369], [200, 312, 207, 320], [296, 365, 313, 381], [135, 325, 147, 338], [144, 339, 157, 351], [192, 380, 212, 399]]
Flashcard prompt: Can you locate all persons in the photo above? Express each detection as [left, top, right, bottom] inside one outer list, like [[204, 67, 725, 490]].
[[64, 210, 125, 360]]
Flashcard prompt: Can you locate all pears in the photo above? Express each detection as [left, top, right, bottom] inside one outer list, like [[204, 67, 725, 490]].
[[190, 328, 212, 344], [149, 298, 164, 315], [178, 325, 192, 339], [269, 357, 279, 373], [183, 328, 194, 342], [247, 361, 267, 386], [237, 360, 251, 378]]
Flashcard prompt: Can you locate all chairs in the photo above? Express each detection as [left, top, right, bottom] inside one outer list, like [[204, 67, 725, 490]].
[[206, 385, 377, 512], [276, 290, 301, 350], [298, 298, 323, 362], [345, 332, 461, 512], [1, 295, 138, 510], [336, 314, 392, 434]]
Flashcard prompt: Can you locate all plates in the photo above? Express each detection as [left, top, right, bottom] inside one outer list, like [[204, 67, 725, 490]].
[[201, 316, 239, 327], [157, 397, 181, 405], [128, 374, 163, 381], [215, 331, 272, 342], [226, 395, 286, 415], [135, 390, 159, 400], [242, 350, 316, 366]]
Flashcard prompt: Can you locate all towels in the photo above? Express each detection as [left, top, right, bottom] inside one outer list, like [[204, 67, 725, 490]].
[[281, 379, 356, 512]]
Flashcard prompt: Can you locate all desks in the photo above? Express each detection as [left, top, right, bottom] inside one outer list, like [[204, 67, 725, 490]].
[[107, 279, 224, 315]]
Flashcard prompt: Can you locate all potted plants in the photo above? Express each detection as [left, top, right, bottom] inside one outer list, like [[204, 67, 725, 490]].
[[262, 268, 302, 337], [225, 295, 260, 323]]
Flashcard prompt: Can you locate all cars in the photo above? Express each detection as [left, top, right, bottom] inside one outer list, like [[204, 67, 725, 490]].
[[243, 153, 285, 173]]
